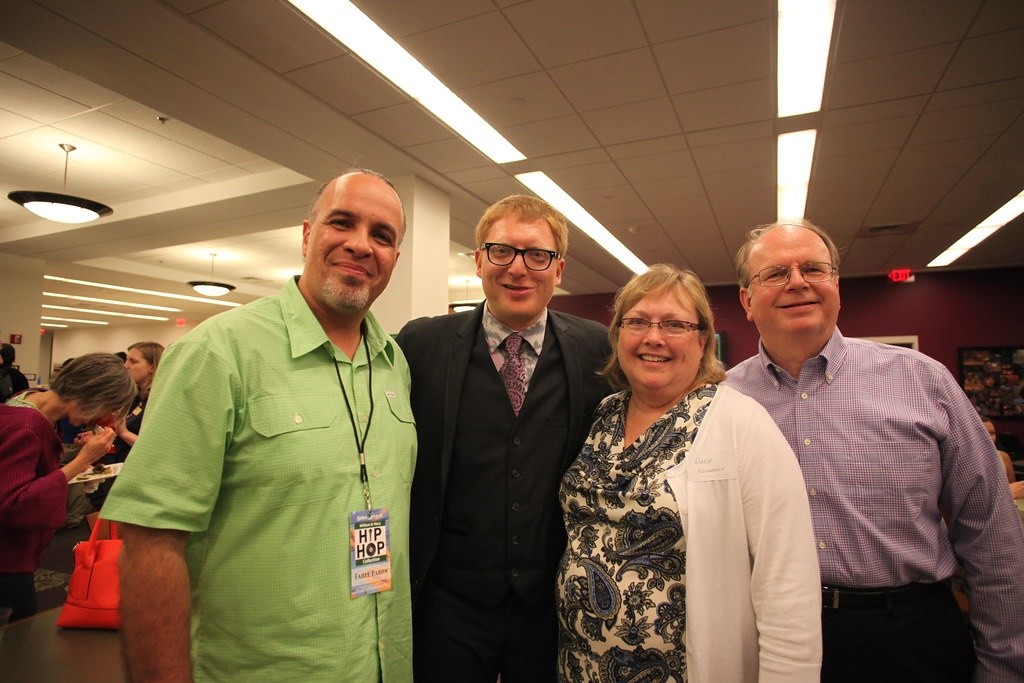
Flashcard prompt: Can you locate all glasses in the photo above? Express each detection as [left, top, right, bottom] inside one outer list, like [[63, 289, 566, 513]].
[[747, 262, 837, 286], [481, 243, 560, 271], [618, 318, 701, 334]]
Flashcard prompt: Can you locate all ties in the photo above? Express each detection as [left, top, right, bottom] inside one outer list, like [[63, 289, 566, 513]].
[[499, 333, 526, 416]]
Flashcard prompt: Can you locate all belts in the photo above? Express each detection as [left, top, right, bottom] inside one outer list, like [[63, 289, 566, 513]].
[[822, 581, 949, 609]]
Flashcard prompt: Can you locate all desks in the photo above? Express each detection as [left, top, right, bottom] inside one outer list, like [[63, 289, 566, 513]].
[[0, 606, 130, 683]]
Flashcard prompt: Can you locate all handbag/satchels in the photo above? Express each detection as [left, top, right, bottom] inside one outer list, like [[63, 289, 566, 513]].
[[56, 516, 123, 630], [0, 369, 13, 401]]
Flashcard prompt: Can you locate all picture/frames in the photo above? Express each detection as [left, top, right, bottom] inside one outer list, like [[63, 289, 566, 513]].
[[956, 346, 1024, 420]]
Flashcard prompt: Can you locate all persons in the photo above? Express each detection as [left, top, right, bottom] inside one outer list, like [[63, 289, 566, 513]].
[[720, 218, 1024, 683], [391, 196, 626, 683], [0, 342, 166, 626], [100, 168, 416, 683], [553, 263, 822, 683], [981, 418, 1024, 500]]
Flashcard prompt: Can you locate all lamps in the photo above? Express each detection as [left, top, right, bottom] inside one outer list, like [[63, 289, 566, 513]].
[[7, 143, 112, 224], [188, 253, 235, 296]]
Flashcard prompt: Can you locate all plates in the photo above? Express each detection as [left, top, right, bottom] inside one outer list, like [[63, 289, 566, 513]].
[[65, 462, 124, 484]]
